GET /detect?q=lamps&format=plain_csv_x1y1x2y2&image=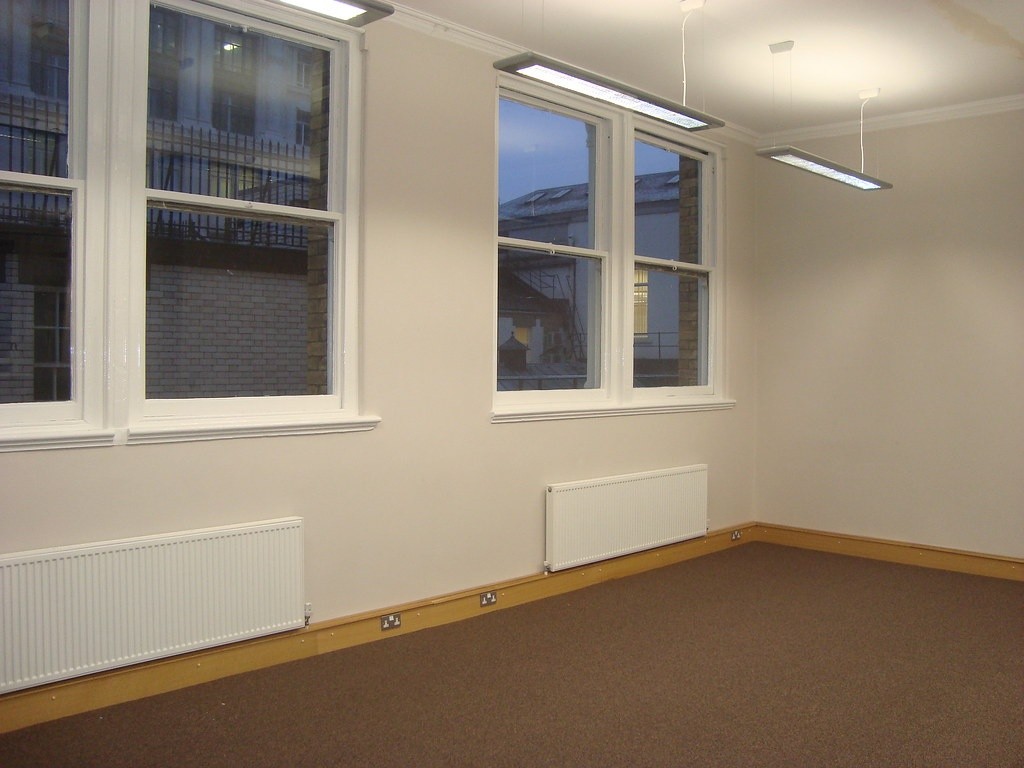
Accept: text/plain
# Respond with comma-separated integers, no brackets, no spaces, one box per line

269,0,895,195
